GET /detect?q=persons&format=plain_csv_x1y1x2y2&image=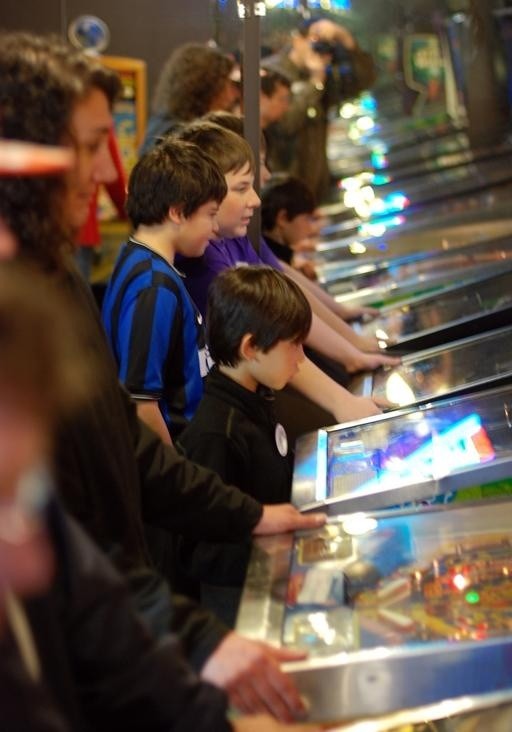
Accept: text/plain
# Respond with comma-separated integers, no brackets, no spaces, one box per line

0,2,403,732
